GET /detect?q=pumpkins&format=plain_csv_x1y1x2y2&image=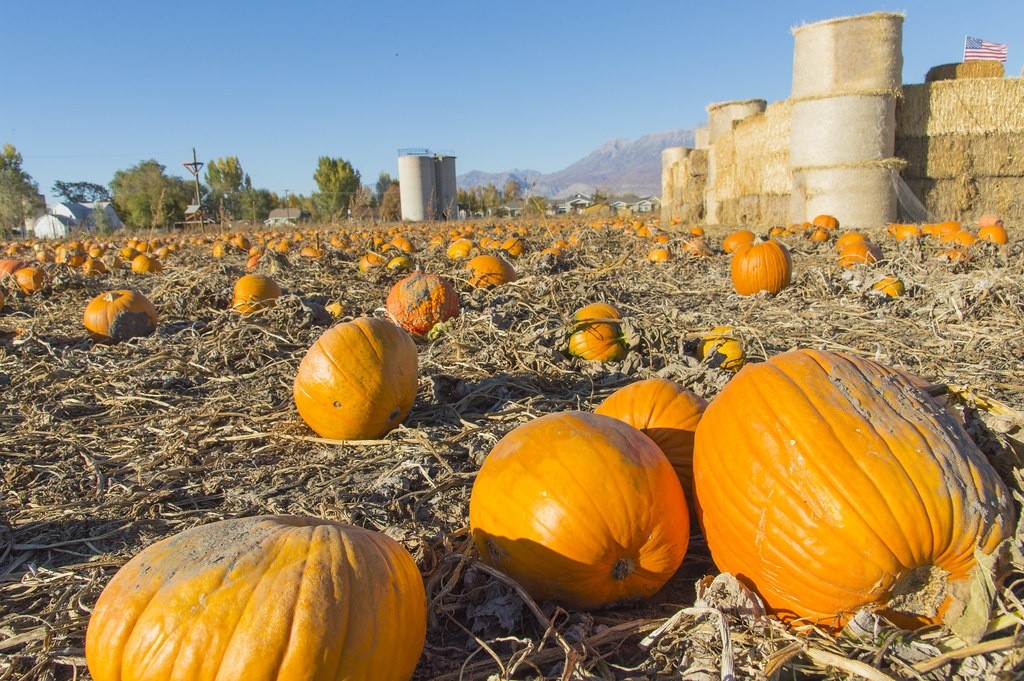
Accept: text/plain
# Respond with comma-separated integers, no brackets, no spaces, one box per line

0,215,1018,681
